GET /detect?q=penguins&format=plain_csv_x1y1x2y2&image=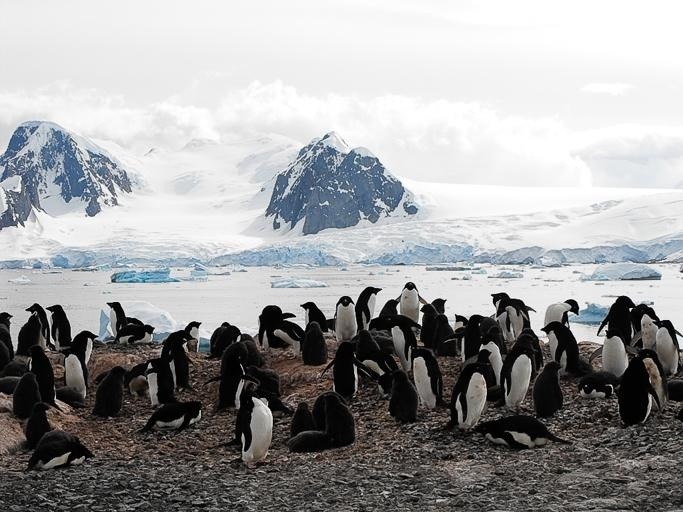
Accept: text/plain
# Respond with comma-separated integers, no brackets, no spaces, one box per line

2,301,202,473
210,283,678,471
